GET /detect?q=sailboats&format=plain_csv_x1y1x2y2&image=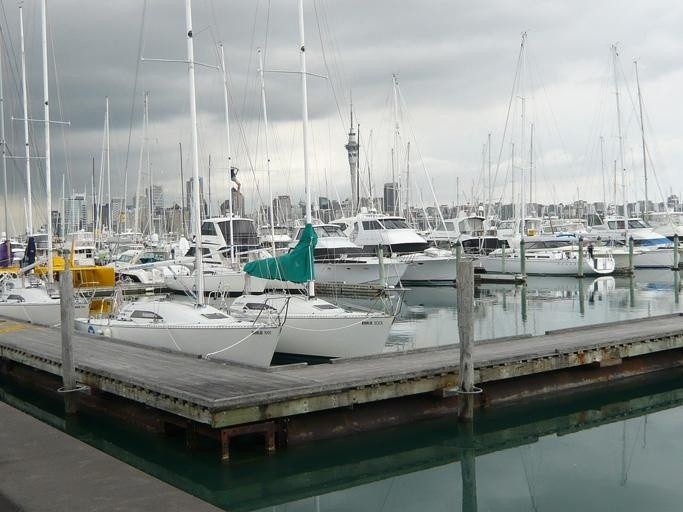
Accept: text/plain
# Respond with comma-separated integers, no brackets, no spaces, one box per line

73,0,292,369
226,1,413,360
0,0,683,334
